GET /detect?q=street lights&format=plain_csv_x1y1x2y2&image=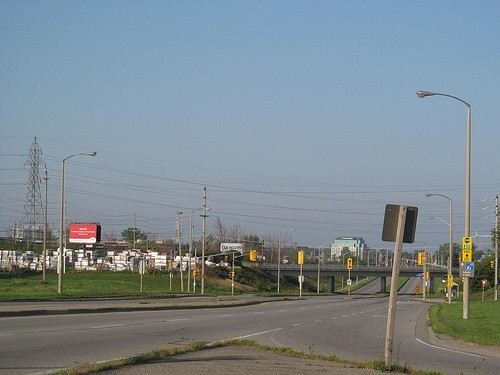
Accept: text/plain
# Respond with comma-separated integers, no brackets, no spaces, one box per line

278,229,296,291
368,249,379,277
427,217,454,305
188,206,211,290
341,249,355,290
425,192,454,305
317,239,335,292
58,151,99,292
356,250,367,286
416,89,473,320
